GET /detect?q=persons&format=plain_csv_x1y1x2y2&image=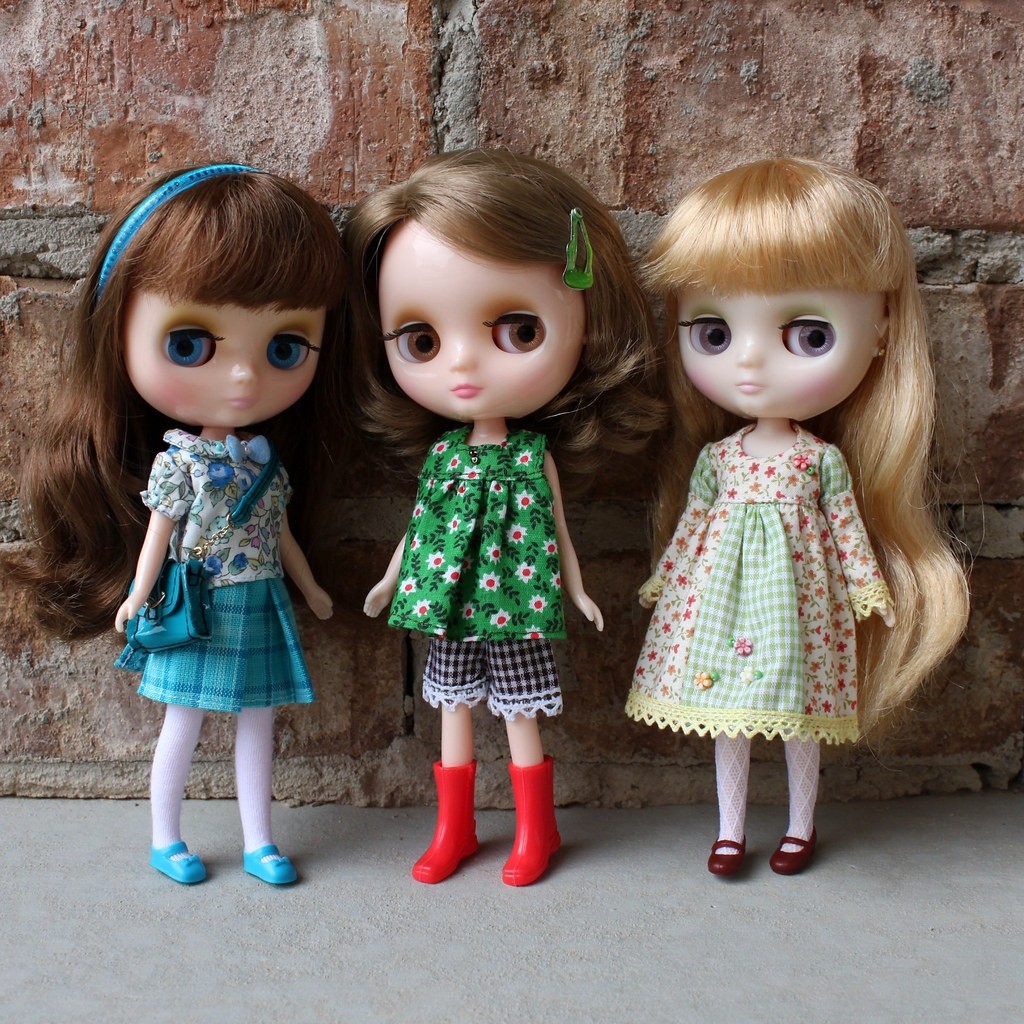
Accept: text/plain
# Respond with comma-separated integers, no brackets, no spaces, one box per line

623,157,972,877
4,158,355,885
343,147,675,887
